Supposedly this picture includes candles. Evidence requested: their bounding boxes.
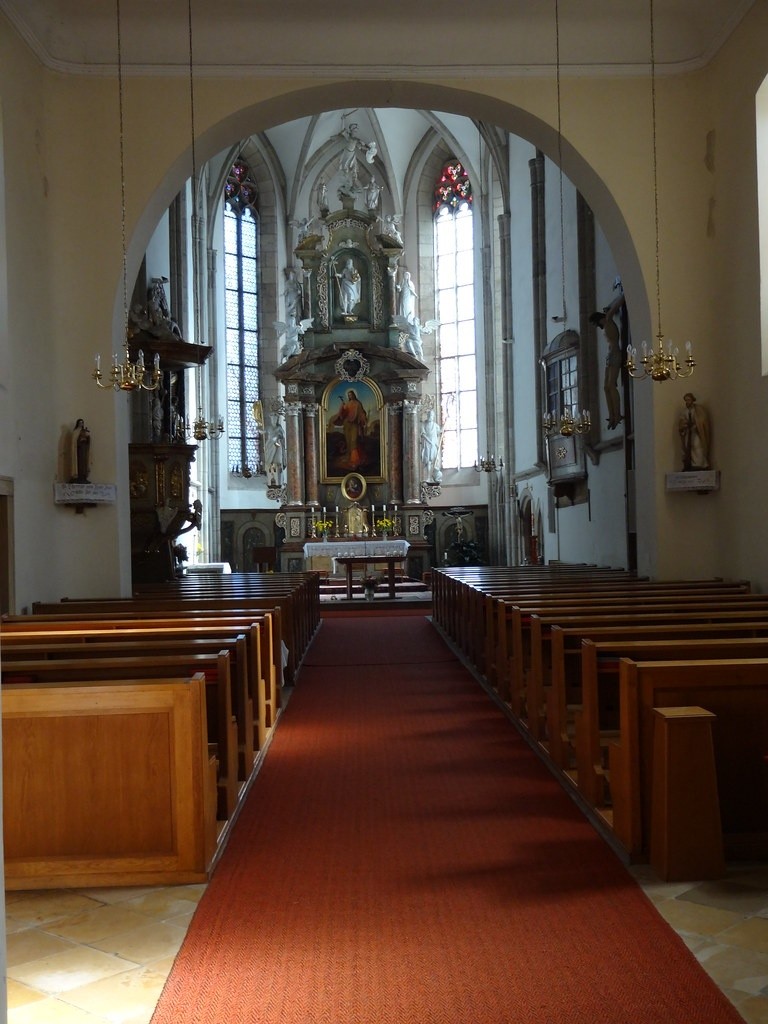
[372,505,374,511]
[336,506,339,512]
[383,505,386,511]
[323,507,326,512]
[395,505,397,510]
[312,507,314,512]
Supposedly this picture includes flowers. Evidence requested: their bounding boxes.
[358,574,379,588]
[376,518,392,531]
[315,521,333,536]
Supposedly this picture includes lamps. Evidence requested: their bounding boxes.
[527,0,590,441]
[624,0,696,381]
[94,0,157,389]
[474,130,505,472]
[172,0,225,444]
[232,143,259,479]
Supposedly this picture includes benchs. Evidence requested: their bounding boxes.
[3,571,320,892]
[429,563,768,866]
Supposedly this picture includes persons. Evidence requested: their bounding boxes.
[589,294,624,430]
[281,272,305,360]
[335,258,361,314]
[395,271,430,363]
[70,418,91,480]
[266,411,285,487]
[421,410,442,484]
[678,392,712,471]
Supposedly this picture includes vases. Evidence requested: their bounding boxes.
[382,532,388,541]
[364,588,374,601]
[322,536,328,543]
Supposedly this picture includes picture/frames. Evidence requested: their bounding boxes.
[319,375,389,485]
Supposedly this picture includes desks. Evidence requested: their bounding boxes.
[335,556,405,599]
[303,540,410,583]
